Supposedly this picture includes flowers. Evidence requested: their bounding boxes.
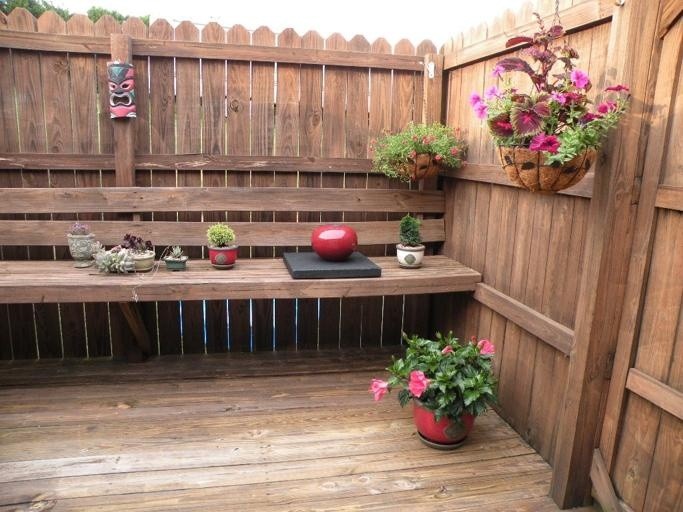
[366,118,474,191]
[367,323,506,442]
[466,1,646,173]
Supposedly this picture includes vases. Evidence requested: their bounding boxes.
[493,137,598,195]
[397,150,443,185]
[413,397,482,453]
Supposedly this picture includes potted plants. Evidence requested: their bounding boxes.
[60,219,241,277]
[394,211,427,270]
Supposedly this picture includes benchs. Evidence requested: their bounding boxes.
[0,174,482,307]
